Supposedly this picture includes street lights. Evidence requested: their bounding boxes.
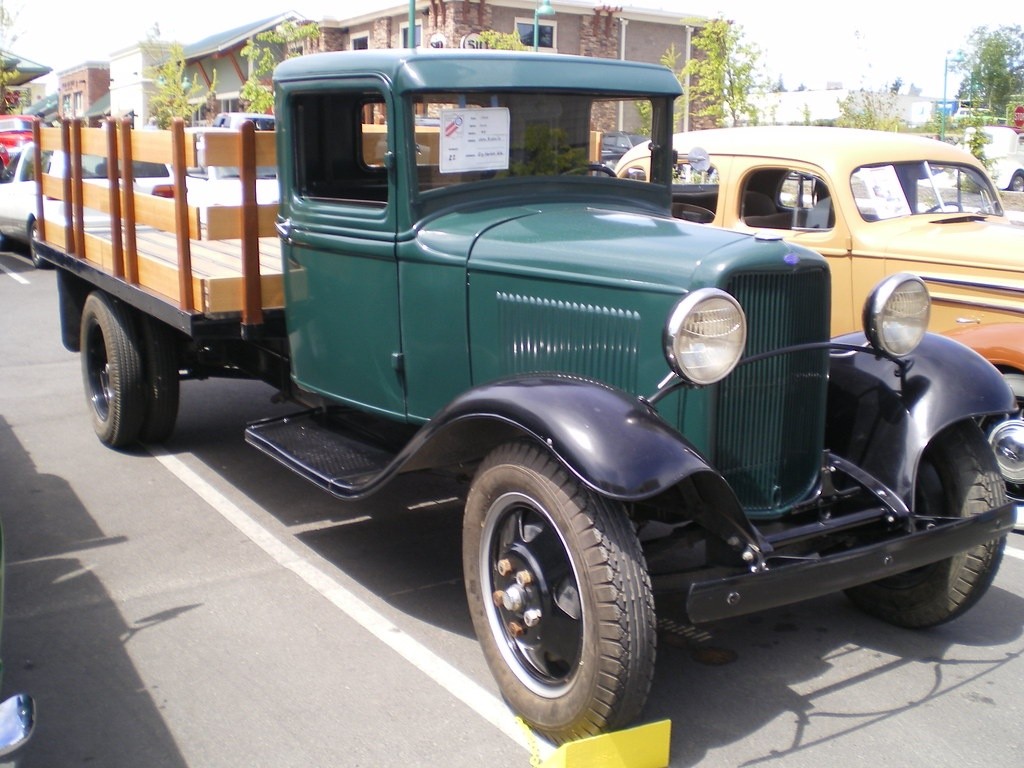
[532,1,554,53]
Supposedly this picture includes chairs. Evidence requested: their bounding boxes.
[744,190,777,215]
[815,174,882,229]
[673,191,716,217]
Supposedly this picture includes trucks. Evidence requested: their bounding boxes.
[23,44,1024,748]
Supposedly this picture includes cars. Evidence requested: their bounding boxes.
[615,124,1024,415]
[1,112,276,270]
[943,106,1023,192]
[597,130,655,180]
